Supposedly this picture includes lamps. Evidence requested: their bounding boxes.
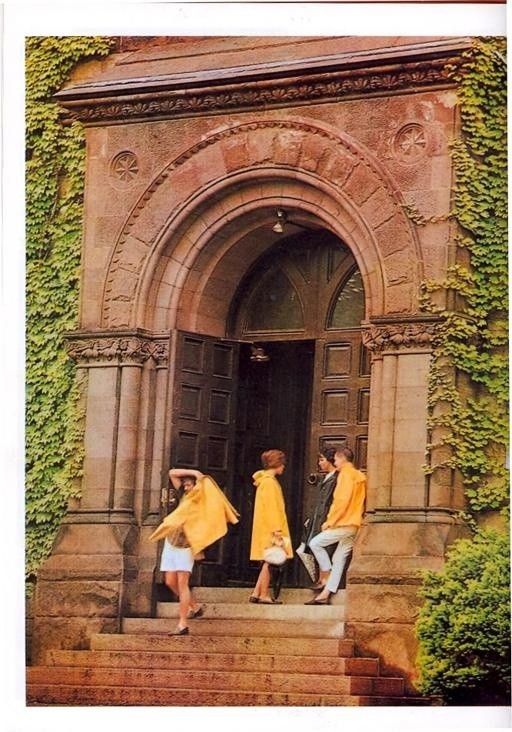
[272,208,289,233]
[246,345,270,363]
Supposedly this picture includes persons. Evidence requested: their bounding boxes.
[304,445,338,581]
[303,448,367,605]
[250,449,294,605]
[149,469,241,635]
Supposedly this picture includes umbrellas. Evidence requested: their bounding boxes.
[269,564,284,600]
[295,527,316,583]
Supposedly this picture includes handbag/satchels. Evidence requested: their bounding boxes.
[263,539,287,566]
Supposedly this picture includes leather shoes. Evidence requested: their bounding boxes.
[168,628,189,636]
[188,608,204,618]
[249,596,261,604]
[304,579,330,605]
[260,598,282,604]
